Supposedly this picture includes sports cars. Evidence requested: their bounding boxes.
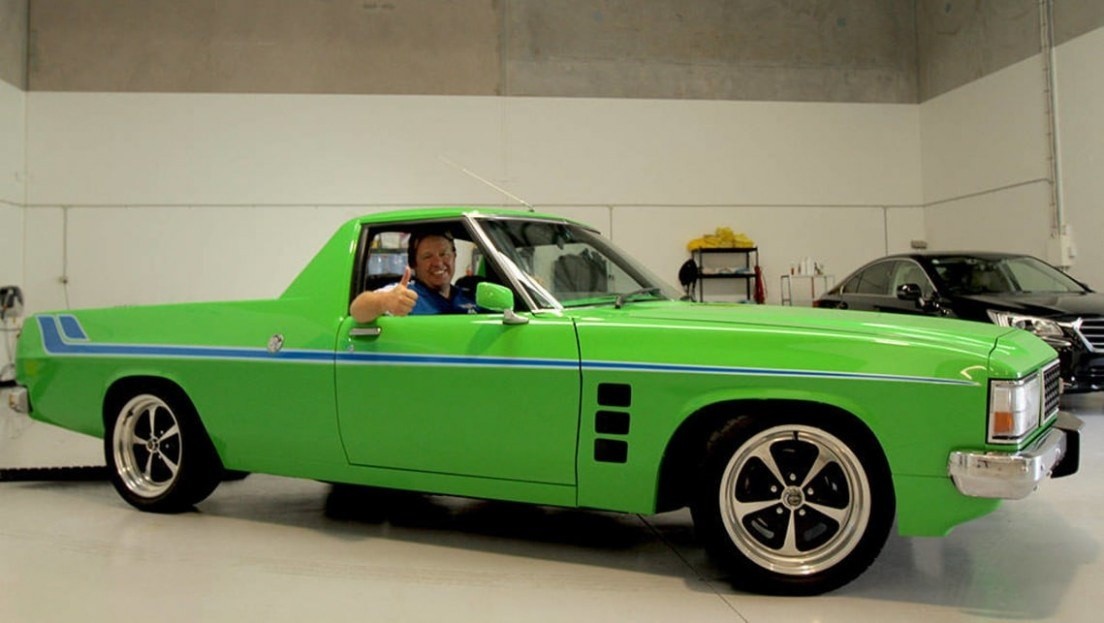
[5,207,1069,598]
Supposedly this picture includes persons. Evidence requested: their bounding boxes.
[350,226,500,324]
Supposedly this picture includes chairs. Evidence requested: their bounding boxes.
[457,275,489,302]
[972,268,1010,293]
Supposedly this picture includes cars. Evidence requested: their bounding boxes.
[810,250,1104,397]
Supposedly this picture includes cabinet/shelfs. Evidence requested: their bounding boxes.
[0,285,24,388]
[690,247,760,304]
[780,274,835,307]
